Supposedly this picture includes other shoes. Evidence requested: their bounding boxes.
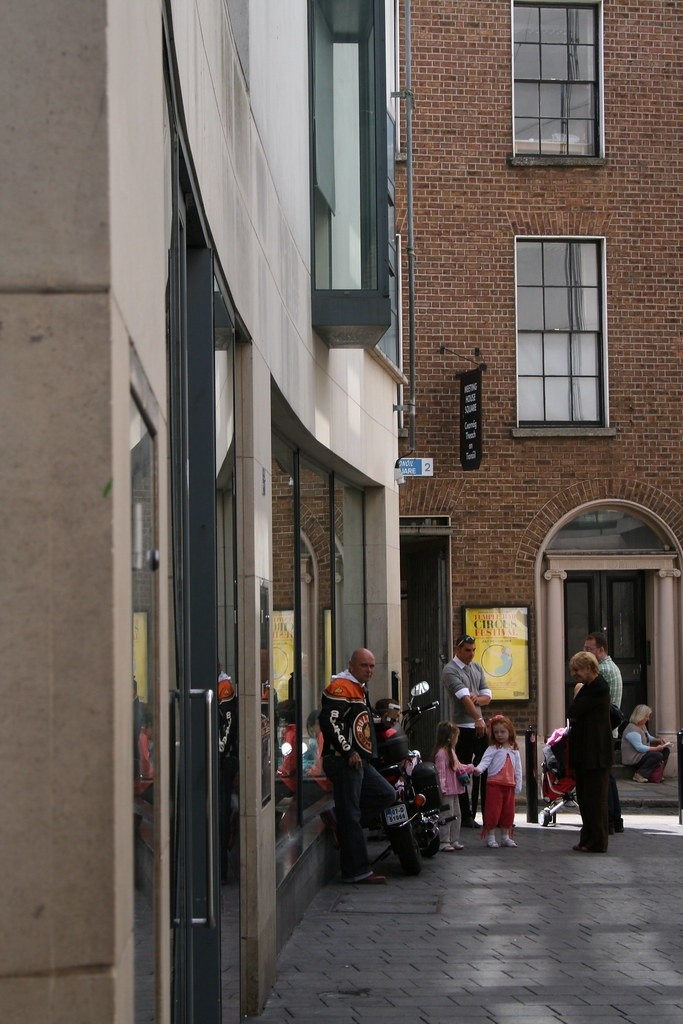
[502,839,518,847]
[607,829,615,835]
[443,846,455,851]
[461,820,481,828]
[452,843,464,848]
[488,841,499,847]
[616,827,624,833]
[633,773,648,783]
[573,844,589,852]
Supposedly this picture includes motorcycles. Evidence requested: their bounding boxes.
[373,680,458,875]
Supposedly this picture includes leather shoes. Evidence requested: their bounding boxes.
[320,810,340,851]
[358,872,386,884]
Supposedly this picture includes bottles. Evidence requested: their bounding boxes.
[456,767,472,787]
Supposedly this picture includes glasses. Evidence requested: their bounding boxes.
[457,636,475,647]
[645,732,649,743]
[583,647,598,652]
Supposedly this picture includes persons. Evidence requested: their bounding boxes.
[566,631,625,855]
[621,705,671,784]
[133,677,155,827]
[275,700,297,807]
[435,633,529,853]
[216,656,241,885]
[319,647,396,885]
[303,710,332,811]
[374,698,402,723]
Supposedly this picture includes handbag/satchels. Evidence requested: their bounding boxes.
[649,761,665,783]
[609,703,625,730]
